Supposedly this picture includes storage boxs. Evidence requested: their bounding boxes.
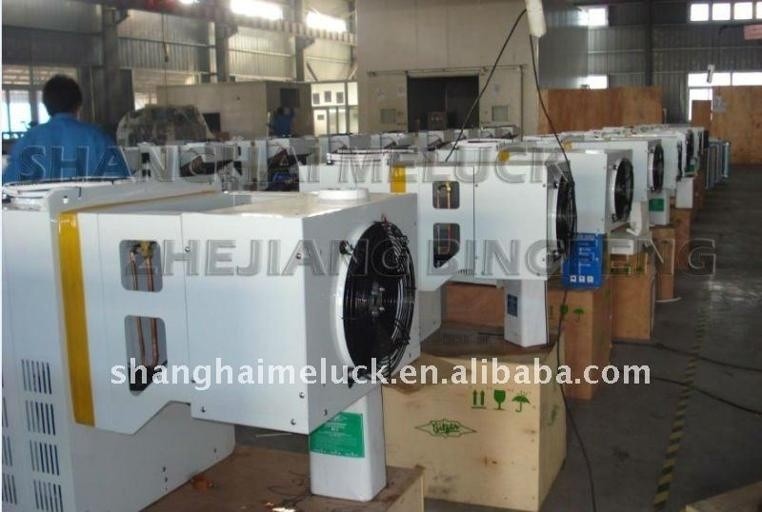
[383,334,568,512]
[441,279,613,400]
[137,445,423,511]
[611,203,691,343]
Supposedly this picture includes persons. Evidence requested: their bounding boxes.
[271,105,294,137]
[2,76,129,184]
